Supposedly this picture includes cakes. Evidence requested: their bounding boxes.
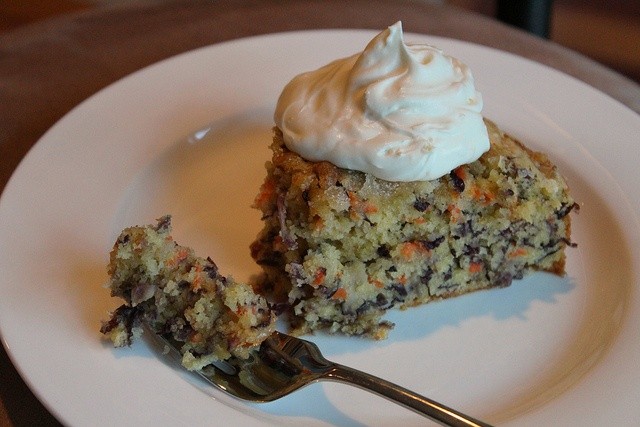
[249,20,580,340]
[98,215,278,371]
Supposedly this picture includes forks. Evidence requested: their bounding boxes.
[139,325,489,423]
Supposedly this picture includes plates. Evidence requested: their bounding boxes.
[1,27,635,426]
